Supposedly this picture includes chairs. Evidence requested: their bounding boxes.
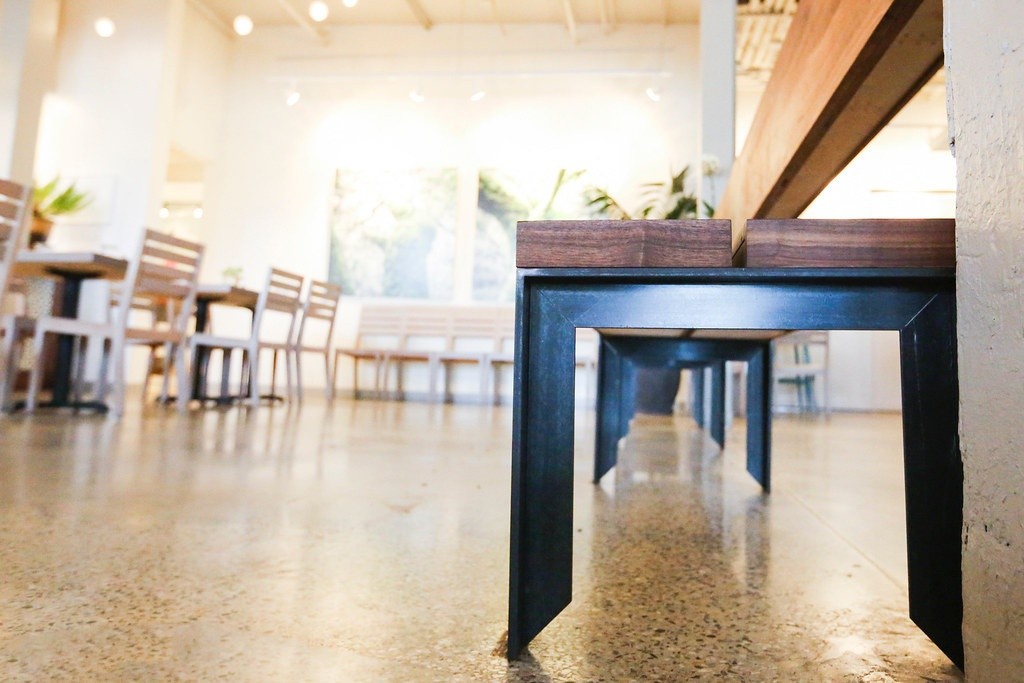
[777,331,827,415]
[0,178,586,422]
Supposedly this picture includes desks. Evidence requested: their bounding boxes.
[189,286,258,408]
[11,251,128,414]
